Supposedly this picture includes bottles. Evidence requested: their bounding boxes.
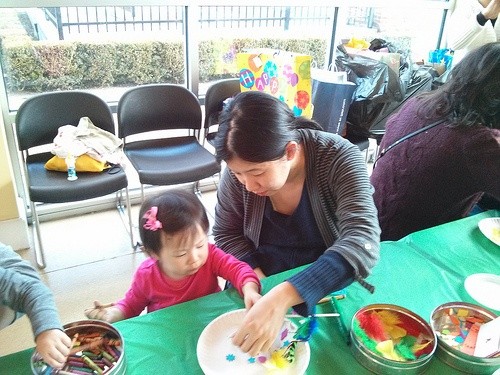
[65,151,78,181]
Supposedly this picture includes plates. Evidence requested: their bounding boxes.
[463,272,500,311]
[478,217,500,246]
[197,309,310,375]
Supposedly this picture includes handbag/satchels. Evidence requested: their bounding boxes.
[234,46,315,119]
[308,59,356,134]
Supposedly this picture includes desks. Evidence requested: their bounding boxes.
[1,204,500,375]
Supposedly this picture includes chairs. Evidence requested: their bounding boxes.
[14,78,243,270]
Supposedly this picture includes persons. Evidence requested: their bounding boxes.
[85,188,263,323]
[212,91,380,355]
[0,241,72,369]
[448,0,500,72]
[370,41,500,242]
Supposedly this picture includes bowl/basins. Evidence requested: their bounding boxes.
[31,318,127,375]
[349,303,438,375]
[430,301,500,375]
